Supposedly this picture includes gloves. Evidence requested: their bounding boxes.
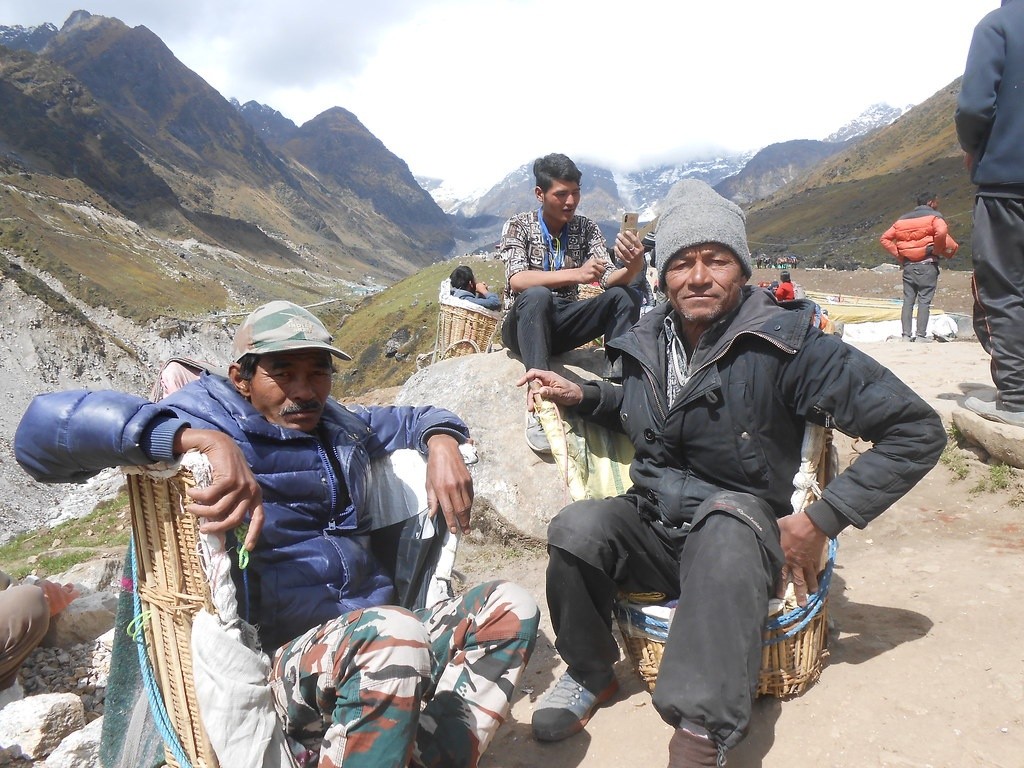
[34,579,79,616]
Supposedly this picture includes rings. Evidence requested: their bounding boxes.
[629,247,635,251]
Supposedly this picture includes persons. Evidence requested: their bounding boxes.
[880,192,959,342]
[0,572,78,690]
[607,247,668,314]
[955,0,1024,427]
[757,254,797,269]
[517,178,948,768]
[450,266,501,310]
[500,153,645,454]
[768,271,797,301]
[15,299,540,768]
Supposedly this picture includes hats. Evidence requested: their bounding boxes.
[231,299,352,361]
[654,177,752,291]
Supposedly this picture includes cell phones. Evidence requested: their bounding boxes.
[620,213,638,245]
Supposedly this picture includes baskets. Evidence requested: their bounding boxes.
[436,295,502,357]
[614,561,828,697]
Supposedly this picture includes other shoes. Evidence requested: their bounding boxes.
[915,335,934,343]
[903,335,910,338]
[964,386,1024,427]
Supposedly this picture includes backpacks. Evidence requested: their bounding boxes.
[792,281,803,299]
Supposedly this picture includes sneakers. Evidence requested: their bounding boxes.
[525,406,552,455]
[532,664,620,742]
